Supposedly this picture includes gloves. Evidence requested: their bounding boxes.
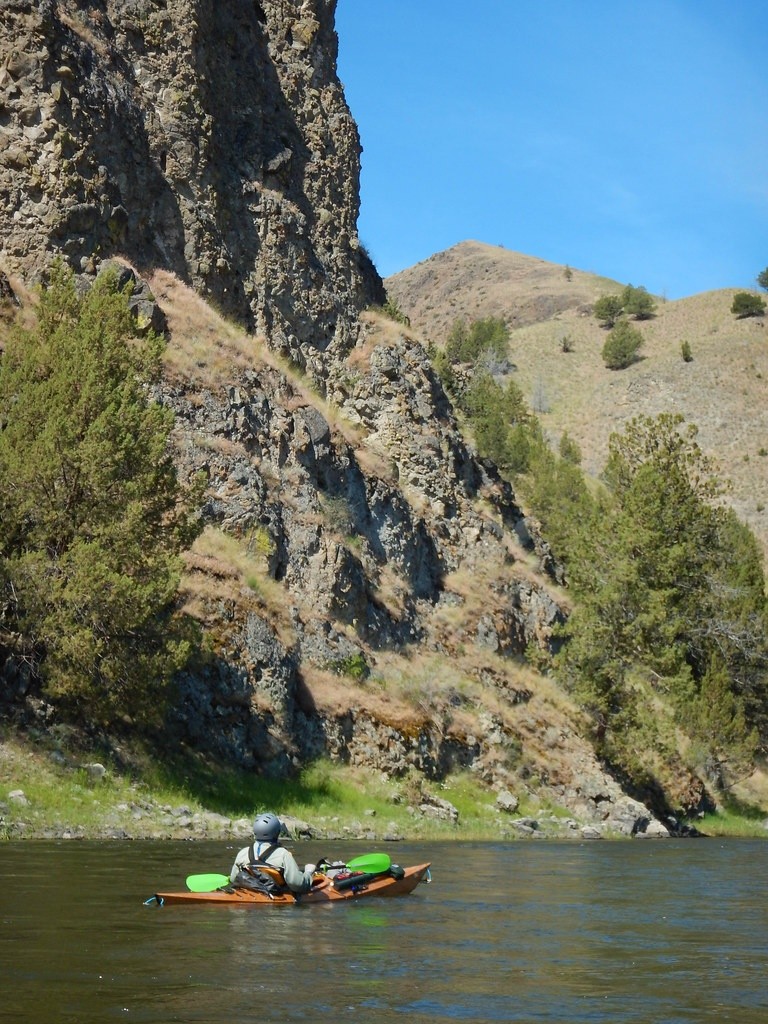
[304,863,317,874]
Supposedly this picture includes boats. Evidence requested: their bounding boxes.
[154,861,433,906]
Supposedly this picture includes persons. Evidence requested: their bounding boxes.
[230,813,317,893]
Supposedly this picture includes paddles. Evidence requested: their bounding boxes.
[185,853,391,893]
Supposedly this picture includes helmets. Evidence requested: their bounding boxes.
[253,813,280,840]
